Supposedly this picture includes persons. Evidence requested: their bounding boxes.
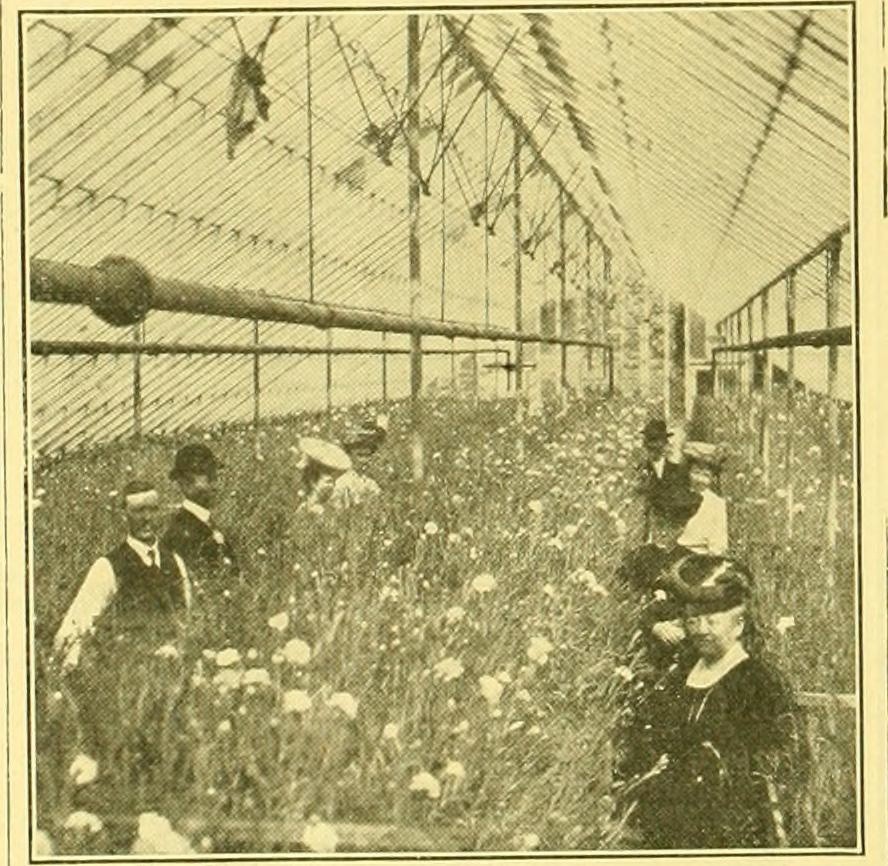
[54,436,380,665]
[630,419,809,849]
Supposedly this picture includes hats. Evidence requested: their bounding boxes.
[682,441,722,465]
[671,550,746,601]
[168,444,224,476]
[641,419,675,438]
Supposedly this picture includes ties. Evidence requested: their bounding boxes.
[149,551,161,573]
